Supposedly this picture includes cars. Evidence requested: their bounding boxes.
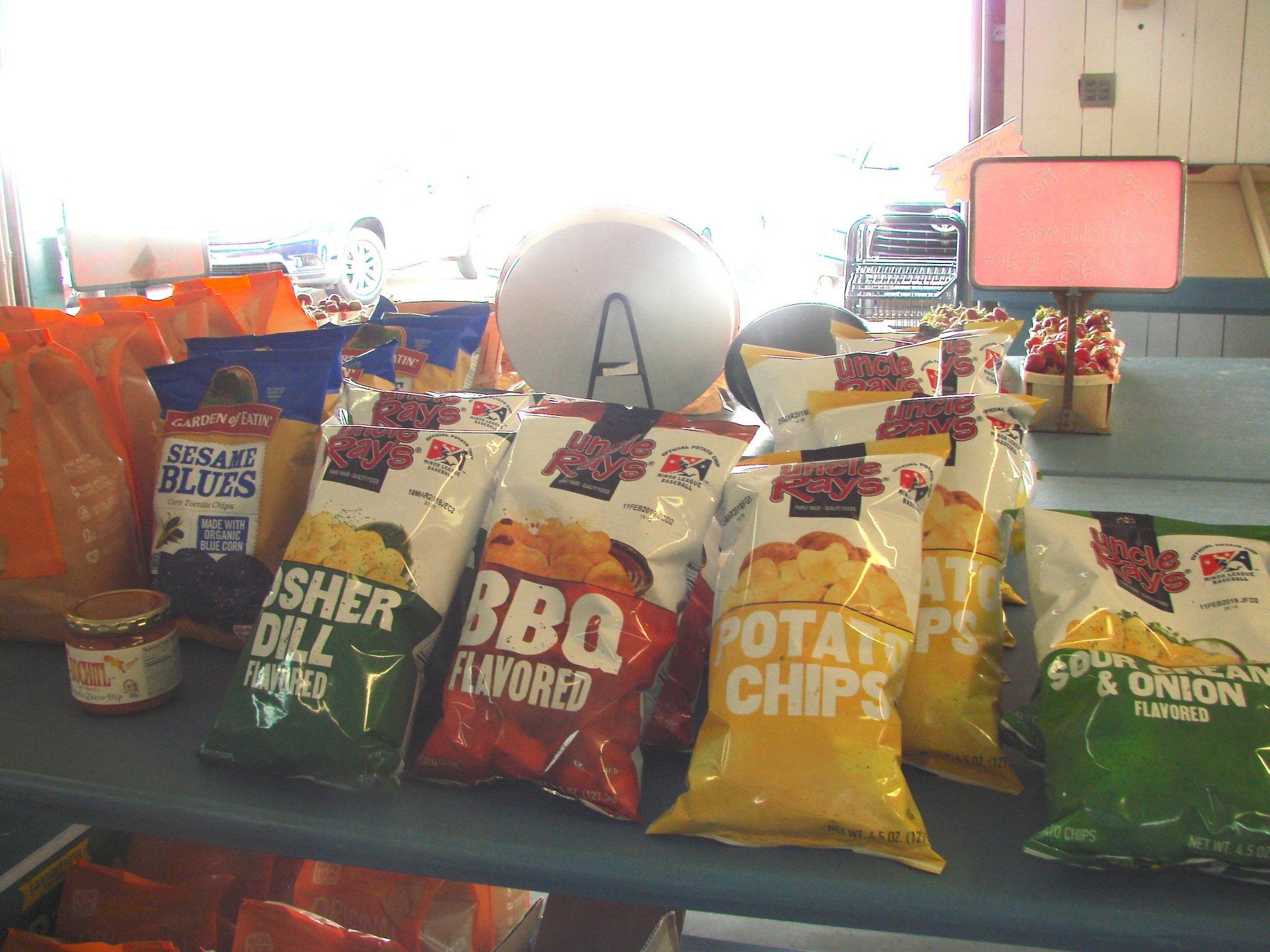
[27,168,511,309]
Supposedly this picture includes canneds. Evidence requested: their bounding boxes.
[62,588,183,715]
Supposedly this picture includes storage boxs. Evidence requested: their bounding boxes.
[1023,339,1125,436]
[492,890,687,952]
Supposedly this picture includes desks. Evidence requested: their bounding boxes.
[0,474,1269,952]
[682,355,1270,482]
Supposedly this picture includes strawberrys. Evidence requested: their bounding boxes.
[924,303,1009,330]
[1023,306,1121,375]
